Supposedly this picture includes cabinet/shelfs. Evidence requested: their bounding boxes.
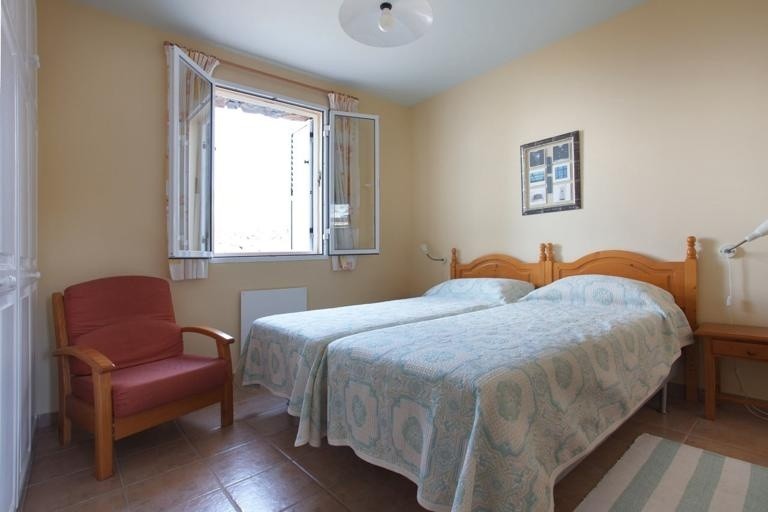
[0,3,37,512]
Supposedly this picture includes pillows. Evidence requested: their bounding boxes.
[524,274,676,310]
[420,275,533,304]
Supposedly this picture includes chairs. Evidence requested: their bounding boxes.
[49,274,238,479]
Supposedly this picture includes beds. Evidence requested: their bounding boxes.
[238,241,544,454]
[326,234,697,512]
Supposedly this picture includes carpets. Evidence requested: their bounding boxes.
[567,431,767,512]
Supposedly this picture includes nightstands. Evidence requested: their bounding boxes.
[692,321,766,421]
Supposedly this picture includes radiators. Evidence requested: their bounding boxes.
[240,286,309,356]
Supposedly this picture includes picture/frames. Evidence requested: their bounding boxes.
[520,128,582,216]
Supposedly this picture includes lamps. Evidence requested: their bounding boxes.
[339,0,432,49]
